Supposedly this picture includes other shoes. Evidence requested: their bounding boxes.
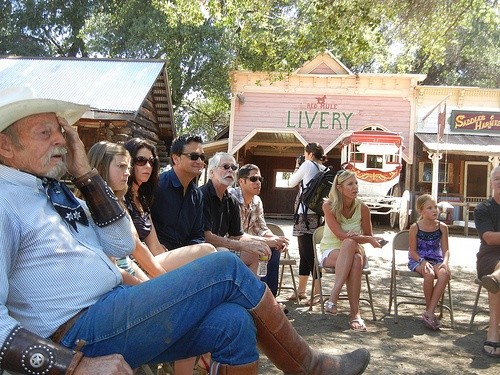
[286,293,307,301]
[305,297,323,306]
[196,352,211,374]
[423,314,442,330]
[279,304,289,315]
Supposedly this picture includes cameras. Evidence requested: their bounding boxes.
[298,155,305,165]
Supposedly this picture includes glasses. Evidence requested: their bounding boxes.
[181,152,205,160]
[215,164,238,170]
[135,156,158,166]
[242,176,263,182]
[336,170,345,185]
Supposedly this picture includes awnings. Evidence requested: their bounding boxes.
[414,133,500,157]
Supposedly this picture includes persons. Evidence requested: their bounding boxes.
[285,141,332,306]
[472,167,500,358]
[0,106,371,375]
[320,170,384,332]
[73,135,289,375]
[407,193,451,332]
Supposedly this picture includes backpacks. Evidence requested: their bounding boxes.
[300,160,337,216]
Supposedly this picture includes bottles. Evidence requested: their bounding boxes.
[256,251,269,277]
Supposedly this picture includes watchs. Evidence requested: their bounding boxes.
[419,258,425,265]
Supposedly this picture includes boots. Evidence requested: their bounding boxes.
[247,282,371,375]
[210,358,261,375]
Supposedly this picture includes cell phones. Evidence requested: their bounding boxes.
[379,240,389,246]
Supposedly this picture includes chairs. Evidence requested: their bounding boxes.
[309,226,377,322]
[388,230,455,328]
[266,224,302,307]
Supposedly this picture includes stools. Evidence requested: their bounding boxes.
[470,279,492,332]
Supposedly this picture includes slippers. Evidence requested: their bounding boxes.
[348,318,366,332]
[480,275,500,294]
[483,337,500,358]
[325,300,338,315]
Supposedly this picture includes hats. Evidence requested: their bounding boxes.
[0,86,91,134]
